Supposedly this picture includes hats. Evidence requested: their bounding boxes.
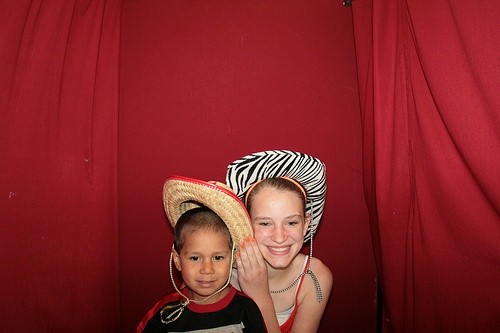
[226,150,327,242]
[163,175,255,270]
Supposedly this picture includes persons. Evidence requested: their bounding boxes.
[135,176,267,333]
[181,150,333,333]
